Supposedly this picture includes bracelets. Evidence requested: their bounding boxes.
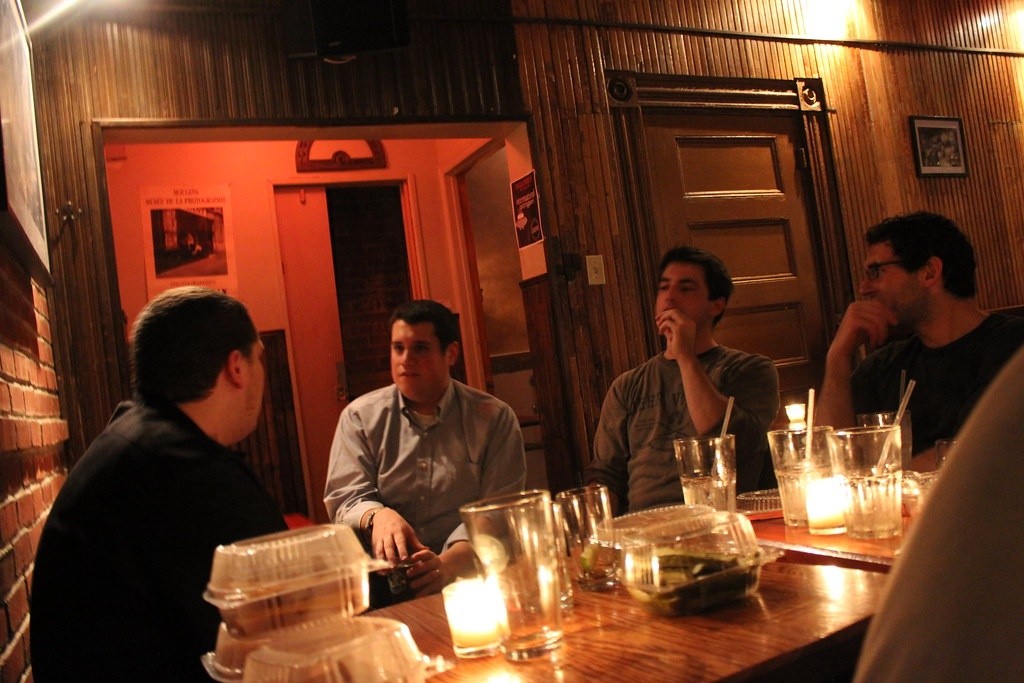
[366,507,387,535]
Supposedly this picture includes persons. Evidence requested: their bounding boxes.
[323,299,528,603]
[579,246,781,519]
[812,210,1024,475]
[28,285,290,683]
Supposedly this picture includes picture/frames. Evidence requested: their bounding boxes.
[908,114,970,179]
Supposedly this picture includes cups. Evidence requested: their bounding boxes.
[458,494,564,659]
[857,409,912,472]
[675,434,736,514]
[769,427,837,527]
[512,505,573,611]
[827,427,903,538]
[556,485,614,591]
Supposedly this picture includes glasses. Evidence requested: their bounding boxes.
[861,260,904,282]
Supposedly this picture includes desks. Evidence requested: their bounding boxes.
[749,513,912,575]
[352,562,889,683]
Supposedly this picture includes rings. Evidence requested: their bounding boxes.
[436,569,440,577]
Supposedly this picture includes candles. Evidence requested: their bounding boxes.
[804,487,848,535]
[441,574,509,657]
